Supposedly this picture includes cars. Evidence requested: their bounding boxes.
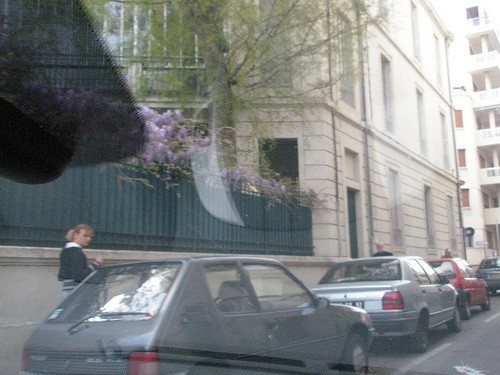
[21,256,374,375]
[311,256,462,353]
[422,258,492,319]
[477,259,500,295]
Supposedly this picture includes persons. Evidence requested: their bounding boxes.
[373,242,394,257]
[58,223,104,302]
[440,247,454,258]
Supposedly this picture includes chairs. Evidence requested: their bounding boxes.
[214,278,255,315]
[354,270,371,279]
